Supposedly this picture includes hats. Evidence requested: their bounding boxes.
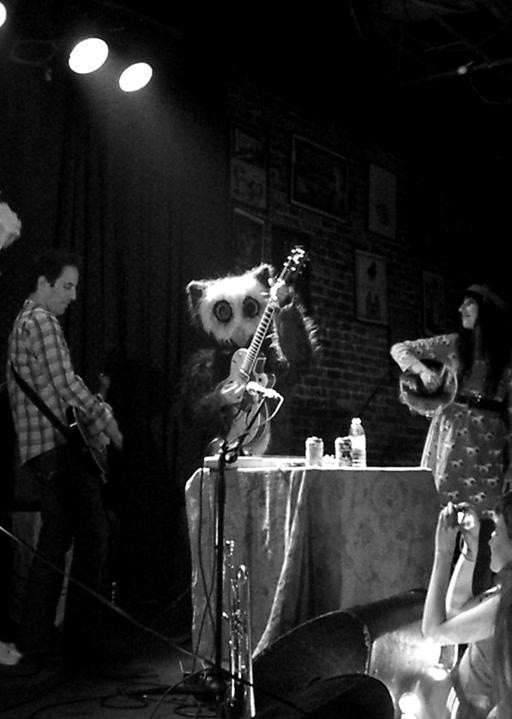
[464,279,503,314]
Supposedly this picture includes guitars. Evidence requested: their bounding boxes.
[213,244,311,447]
[65,374,111,478]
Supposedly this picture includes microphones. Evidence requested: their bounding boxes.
[246,381,281,399]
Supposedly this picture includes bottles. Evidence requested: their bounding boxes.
[348,417,367,467]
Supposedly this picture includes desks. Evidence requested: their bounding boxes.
[186,465,442,682]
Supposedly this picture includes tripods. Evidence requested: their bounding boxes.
[127,395,269,719]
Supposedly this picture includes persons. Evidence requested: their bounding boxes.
[369,493,512,719]
[389,281,509,597]
[7,252,154,683]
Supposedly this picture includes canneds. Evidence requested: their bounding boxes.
[335,437,352,464]
[304,437,324,464]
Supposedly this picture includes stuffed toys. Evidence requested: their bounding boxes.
[176,262,320,495]
